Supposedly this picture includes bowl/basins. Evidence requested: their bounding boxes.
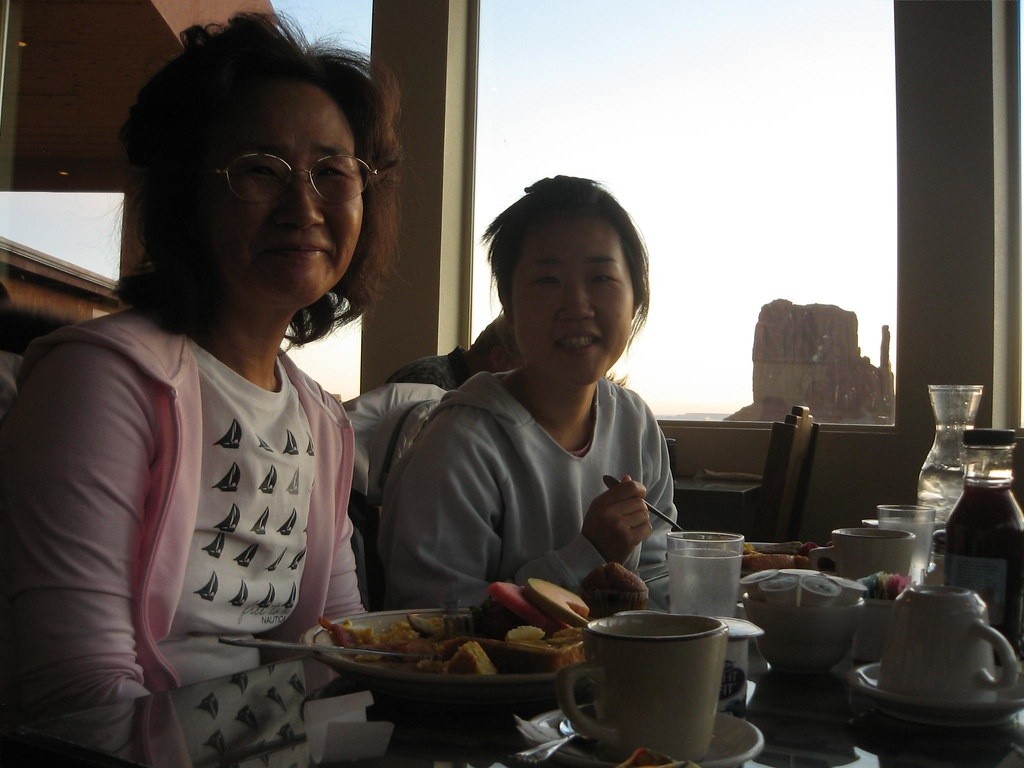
[742,593,866,675]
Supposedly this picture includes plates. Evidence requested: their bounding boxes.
[525,710,763,768]
[300,610,556,706]
[741,543,777,576]
[847,664,1024,727]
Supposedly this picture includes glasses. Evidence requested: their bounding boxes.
[202,154,379,203]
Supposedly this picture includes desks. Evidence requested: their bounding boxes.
[668,473,765,511]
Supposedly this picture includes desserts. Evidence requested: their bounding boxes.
[577,562,650,619]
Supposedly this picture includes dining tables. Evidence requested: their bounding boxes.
[0,553,1024,768]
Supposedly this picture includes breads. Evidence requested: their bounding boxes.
[445,640,498,676]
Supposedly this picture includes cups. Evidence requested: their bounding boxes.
[558,615,729,760]
[877,504,935,586]
[878,584,1018,700]
[810,527,917,581]
[717,617,763,719]
[666,531,744,618]
[852,598,895,655]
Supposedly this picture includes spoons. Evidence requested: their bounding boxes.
[510,705,598,762]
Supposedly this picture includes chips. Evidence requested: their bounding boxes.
[502,625,547,642]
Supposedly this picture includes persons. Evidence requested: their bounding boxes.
[342,173,679,612]
[0,10,394,721]
[42,656,341,768]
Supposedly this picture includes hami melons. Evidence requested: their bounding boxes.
[525,576,595,628]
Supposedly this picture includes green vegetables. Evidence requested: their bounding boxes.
[469,595,526,639]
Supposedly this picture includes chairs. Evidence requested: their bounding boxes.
[749,407,821,543]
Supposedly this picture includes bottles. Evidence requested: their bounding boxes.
[924,527,947,585]
[945,429,1024,666]
[918,385,985,527]
[665,438,676,482]
